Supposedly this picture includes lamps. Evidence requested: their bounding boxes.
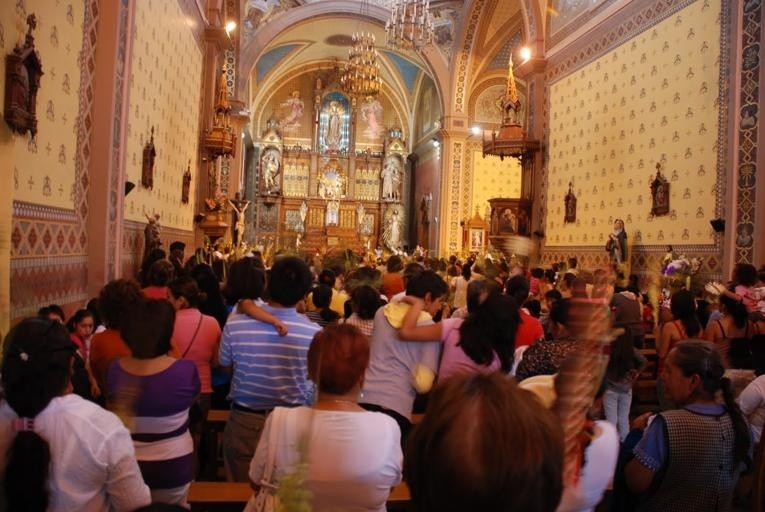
[338,0,385,104]
[382,0,440,53]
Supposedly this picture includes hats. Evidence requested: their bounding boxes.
[2,316,79,352]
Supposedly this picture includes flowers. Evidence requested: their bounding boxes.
[661,244,698,283]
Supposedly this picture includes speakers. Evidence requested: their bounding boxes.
[196,213,205,223]
[125,181,135,196]
[533,231,544,238]
[710,218,725,232]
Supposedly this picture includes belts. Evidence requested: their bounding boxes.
[232,401,272,415]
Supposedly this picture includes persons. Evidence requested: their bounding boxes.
[279,90,304,127]
[324,106,342,146]
[145,213,163,232]
[144,218,163,248]
[361,95,384,135]
[605,219,628,281]
[228,199,250,247]
[2,245,765,512]
[391,209,399,241]
[391,167,400,201]
[261,152,276,188]
[380,164,393,199]
